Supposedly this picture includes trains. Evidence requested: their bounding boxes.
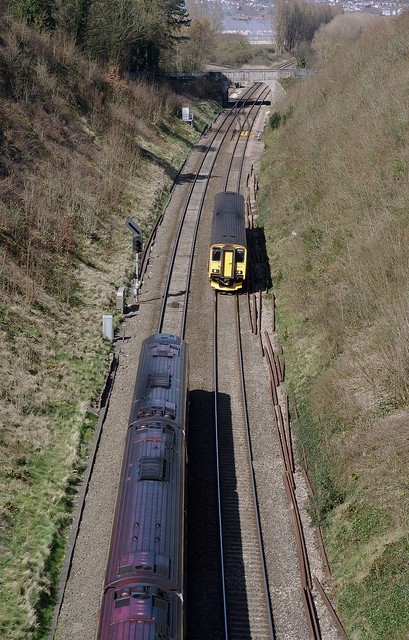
[209,191,247,294]
[98,334,188,640]
